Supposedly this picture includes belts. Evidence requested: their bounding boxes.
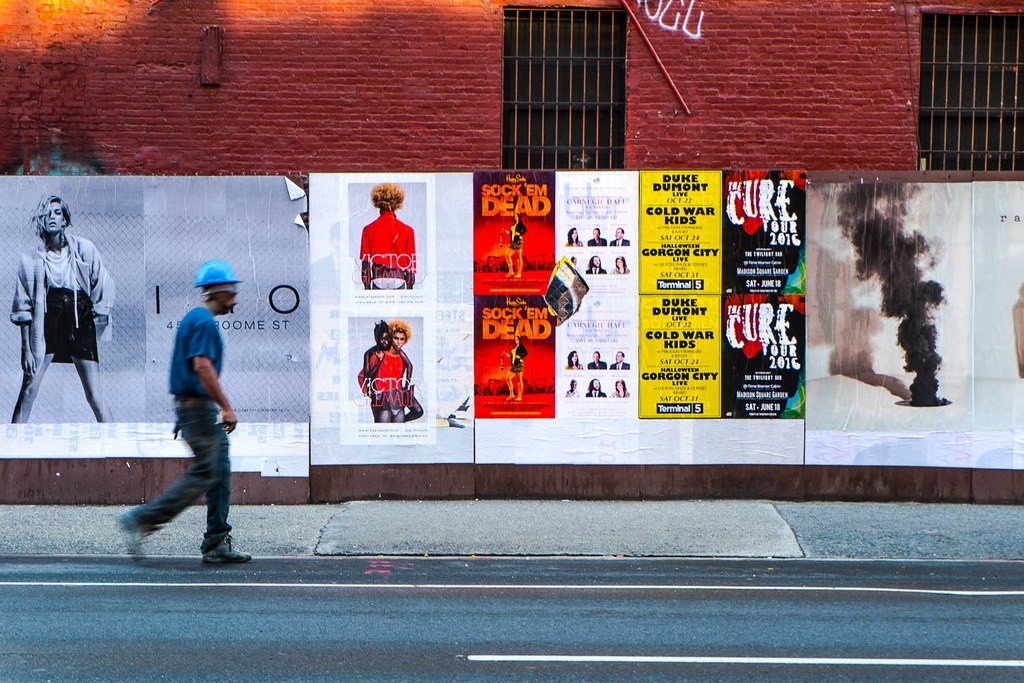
[174,398,215,408]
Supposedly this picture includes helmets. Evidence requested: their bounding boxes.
[194,260,245,287]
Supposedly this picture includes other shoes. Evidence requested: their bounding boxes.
[201,535,251,563]
[118,511,162,564]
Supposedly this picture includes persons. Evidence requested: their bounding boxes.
[357,319,423,423]
[501,212,630,402]
[120,258,251,565]
[9,195,116,424]
[359,183,417,290]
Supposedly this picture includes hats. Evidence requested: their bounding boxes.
[197,283,239,301]
